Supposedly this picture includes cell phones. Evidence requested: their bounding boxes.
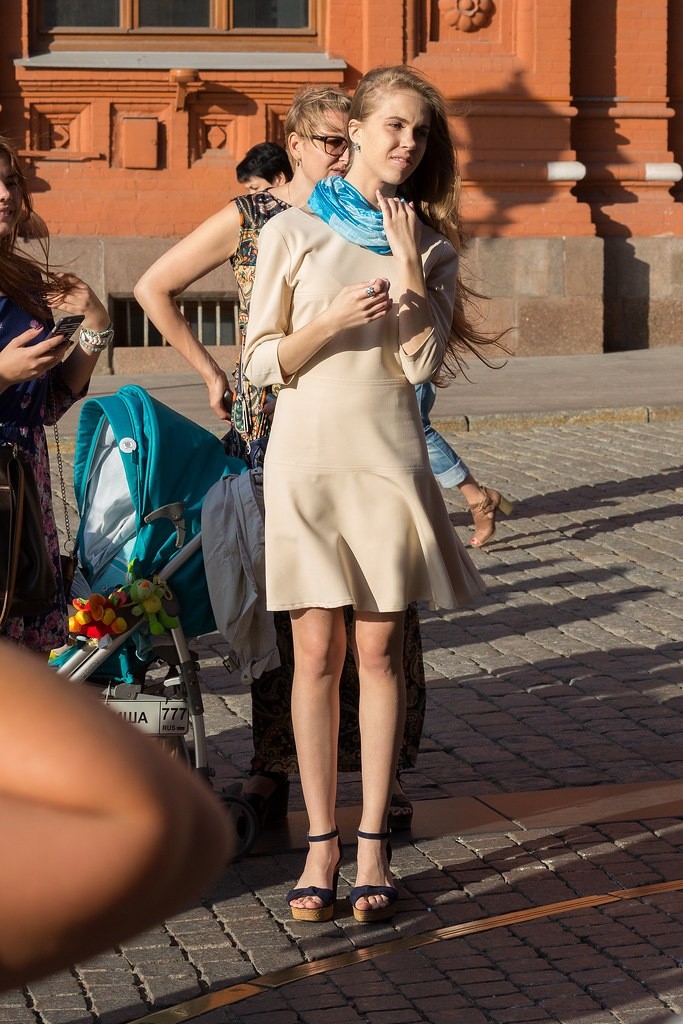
[43,314,85,342]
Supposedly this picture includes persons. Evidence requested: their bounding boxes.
[241,66,488,921]
[0,138,115,652]
[236,143,514,547]
[133,86,427,837]
[0,639,236,991]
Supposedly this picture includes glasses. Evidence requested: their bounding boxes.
[300,134,349,156]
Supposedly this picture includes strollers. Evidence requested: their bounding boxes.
[44,384,257,859]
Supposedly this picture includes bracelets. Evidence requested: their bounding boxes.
[78,322,114,353]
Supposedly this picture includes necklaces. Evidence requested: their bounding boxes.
[288,183,293,206]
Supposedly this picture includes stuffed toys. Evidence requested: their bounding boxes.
[67,557,180,641]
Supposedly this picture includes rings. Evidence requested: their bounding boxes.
[366,286,374,297]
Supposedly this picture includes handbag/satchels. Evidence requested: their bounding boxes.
[0,449,50,633]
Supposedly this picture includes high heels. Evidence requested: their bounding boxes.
[387,772,414,830]
[350,828,399,922]
[469,487,513,547]
[285,827,343,921]
[227,770,290,822]
